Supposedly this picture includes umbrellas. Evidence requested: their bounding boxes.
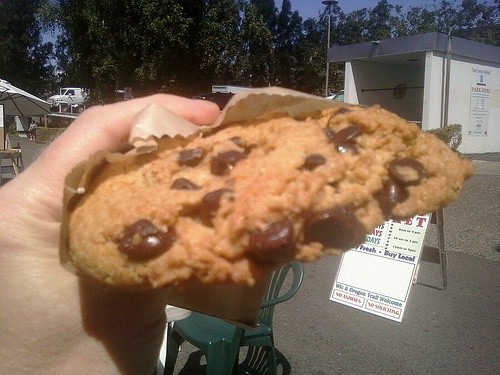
[0,80,52,150]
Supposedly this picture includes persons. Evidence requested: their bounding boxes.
[0,92,220,375]
[27,119,37,142]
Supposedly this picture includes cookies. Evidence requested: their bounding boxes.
[65,102,469,289]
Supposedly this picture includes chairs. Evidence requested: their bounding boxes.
[163,261,304,375]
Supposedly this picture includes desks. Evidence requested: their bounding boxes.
[0,152,25,187]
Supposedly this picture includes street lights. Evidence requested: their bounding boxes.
[322,0,338,98]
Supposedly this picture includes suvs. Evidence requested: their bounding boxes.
[48,87,84,112]
